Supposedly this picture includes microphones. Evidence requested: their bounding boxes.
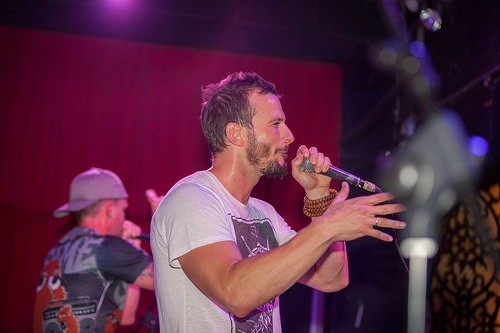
[129,232,150,240]
[299,156,382,194]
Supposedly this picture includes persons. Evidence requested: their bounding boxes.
[32,167,167,333]
[150,71,410,333]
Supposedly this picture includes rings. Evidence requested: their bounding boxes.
[375,217,380,226]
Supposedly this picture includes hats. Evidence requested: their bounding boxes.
[54,167,129,220]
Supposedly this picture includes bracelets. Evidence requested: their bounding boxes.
[303,188,341,218]
